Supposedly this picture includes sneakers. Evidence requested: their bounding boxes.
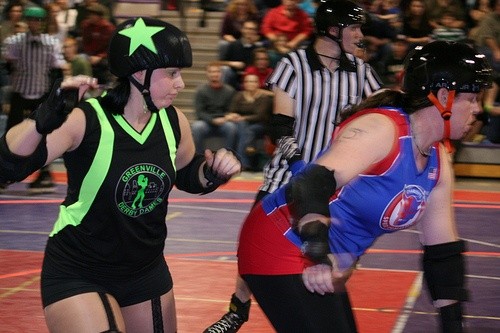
[202,313,244,333]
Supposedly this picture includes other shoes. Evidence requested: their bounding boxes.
[29,171,56,193]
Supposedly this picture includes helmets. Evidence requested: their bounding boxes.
[401,40,493,91]
[315,0,365,32]
[108,16,193,77]
[23,7,48,21]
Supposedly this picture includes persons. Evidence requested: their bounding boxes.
[201,0,385,333]
[0,0,500,191]
[0,16,242,333]
[232,36,497,333]
